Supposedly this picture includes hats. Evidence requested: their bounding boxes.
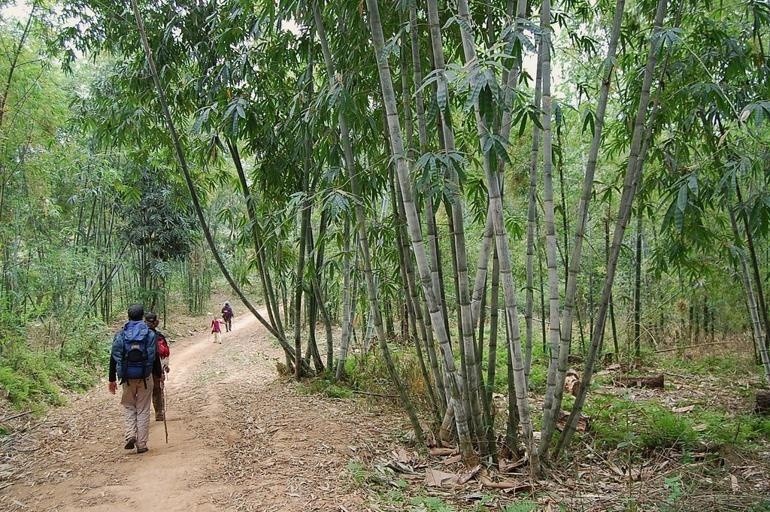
[145,314,157,323]
[128,304,144,319]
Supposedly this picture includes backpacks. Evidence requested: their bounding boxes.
[120,325,150,379]
[223,307,231,317]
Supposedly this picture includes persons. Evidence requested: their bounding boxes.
[210,314,227,344]
[221,302,234,332]
[145,312,171,422]
[108,305,160,453]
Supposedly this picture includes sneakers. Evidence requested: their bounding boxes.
[124,437,137,449]
[213,341,221,344]
[226,329,231,332]
[156,413,164,421]
[137,448,149,453]
